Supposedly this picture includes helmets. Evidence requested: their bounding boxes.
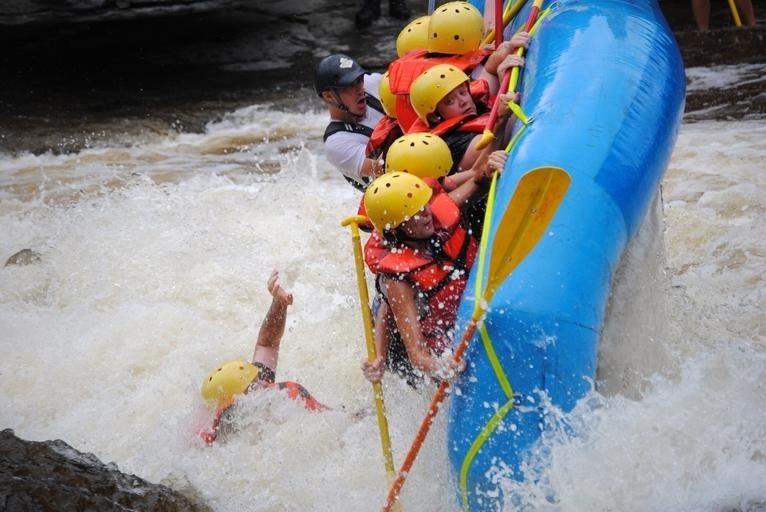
[314,52,365,98]
[363,0,484,236]
[201,359,259,415]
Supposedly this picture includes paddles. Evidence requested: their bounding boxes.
[382,165,572,512]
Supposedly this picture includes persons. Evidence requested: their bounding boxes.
[313,0,534,385]
[692,0,757,32]
[200,269,337,446]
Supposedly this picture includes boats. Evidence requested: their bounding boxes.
[446,0,686,512]
